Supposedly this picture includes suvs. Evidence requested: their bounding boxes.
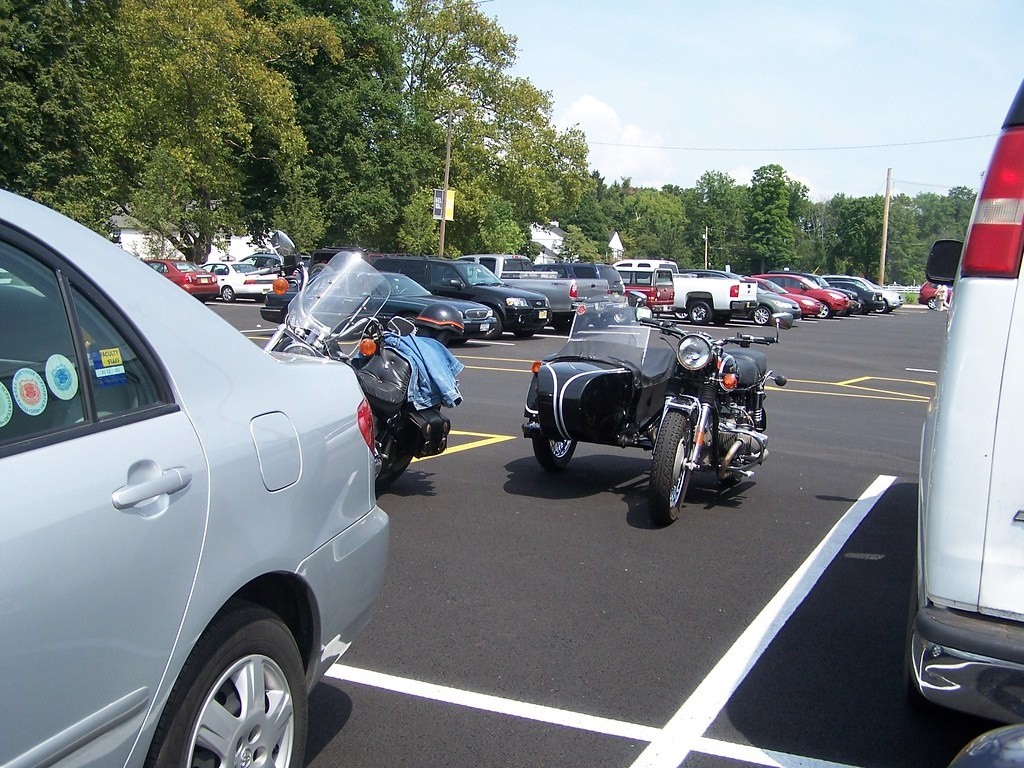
[364,255,555,347]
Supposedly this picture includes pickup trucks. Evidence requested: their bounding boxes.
[453,252,615,327]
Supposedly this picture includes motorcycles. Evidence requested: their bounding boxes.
[517,288,794,526]
[245,228,462,490]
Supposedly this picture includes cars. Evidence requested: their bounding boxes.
[0,184,402,766]
[918,280,953,311]
[146,259,222,308]
[259,269,500,350]
[527,259,909,329]
[193,262,284,303]
